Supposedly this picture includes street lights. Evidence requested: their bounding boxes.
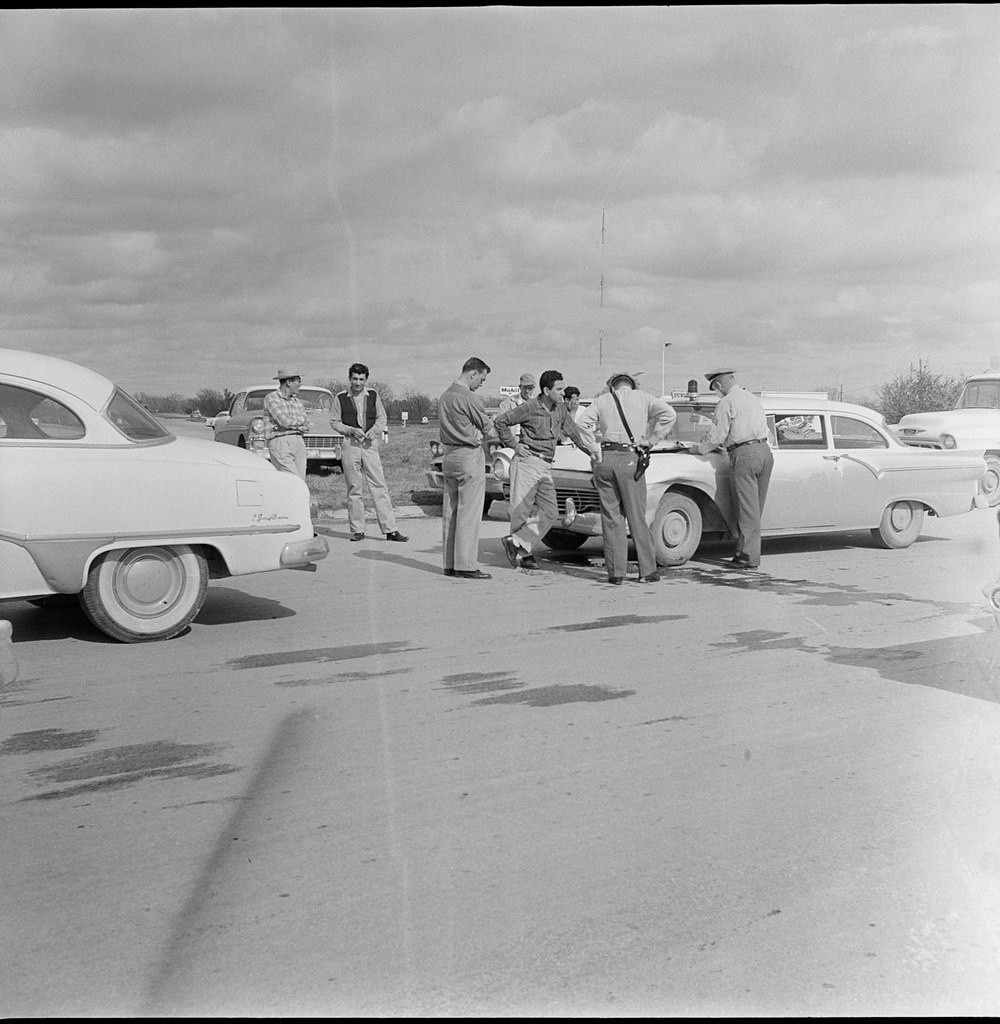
[660,341,676,398]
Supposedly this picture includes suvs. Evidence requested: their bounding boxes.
[870,373,1000,510]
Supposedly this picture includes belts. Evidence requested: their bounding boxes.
[268,433,303,441]
[728,439,767,453]
[532,453,552,464]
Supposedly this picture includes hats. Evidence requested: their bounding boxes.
[519,374,536,385]
[704,370,736,391]
[272,365,305,380]
[607,372,639,391]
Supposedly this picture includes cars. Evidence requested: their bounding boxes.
[0,347,332,643]
[426,384,988,568]
[190,411,202,418]
[205,411,230,430]
[213,385,345,471]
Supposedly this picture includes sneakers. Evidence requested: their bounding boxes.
[517,547,539,569]
[501,535,519,568]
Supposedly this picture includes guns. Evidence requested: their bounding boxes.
[634,449,650,482]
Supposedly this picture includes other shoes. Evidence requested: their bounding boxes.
[608,577,624,584]
[638,573,660,583]
[724,559,758,571]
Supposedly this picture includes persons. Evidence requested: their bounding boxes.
[499,373,537,444]
[690,368,774,570]
[576,371,676,585]
[263,367,311,480]
[493,370,596,570]
[437,357,495,578]
[330,363,410,542]
[557,386,587,446]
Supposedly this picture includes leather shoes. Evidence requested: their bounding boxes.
[350,532,364,541]
[387,531,408,542]
[444,568,491,580]
